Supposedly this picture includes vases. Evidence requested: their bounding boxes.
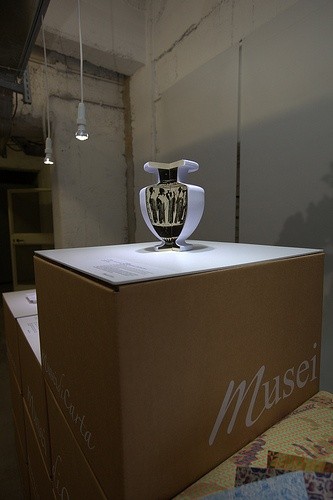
[139,161,203,252]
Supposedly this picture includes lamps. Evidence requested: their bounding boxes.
[74,103,88,140]
[43,137,53,164]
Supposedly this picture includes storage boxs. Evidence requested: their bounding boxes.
[2,237,324,500]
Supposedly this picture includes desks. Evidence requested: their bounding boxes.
[168,389,333,499]
